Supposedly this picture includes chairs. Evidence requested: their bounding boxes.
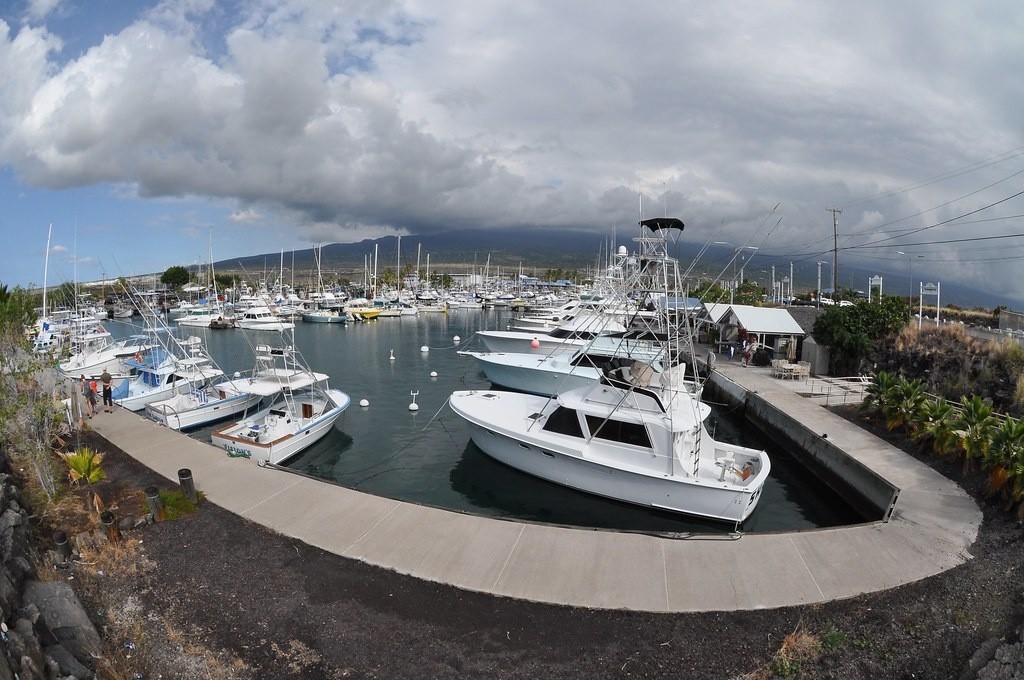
[771,358,811,381]
[730,466,750,480]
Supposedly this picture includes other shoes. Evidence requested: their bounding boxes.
[104,410,109,412]
[92,411,95,413]
[96,412,98,414]
[110,411,112,413]
[89,416,92,419]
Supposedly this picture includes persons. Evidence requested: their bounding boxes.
[531,337,539,348]
[646,303,654,310]
[101,370,113,414]
[728,338,757,360]
[80,374,93,419]
[89,377,98,414]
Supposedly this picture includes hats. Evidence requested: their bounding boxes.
[103,367,107,371]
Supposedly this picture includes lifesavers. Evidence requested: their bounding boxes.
[135,352,142,363]
[706,352,716,364]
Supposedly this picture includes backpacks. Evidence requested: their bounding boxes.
[82,382,92,398]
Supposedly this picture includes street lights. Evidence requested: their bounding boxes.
[819,259,835,293]
[713,242,758,296]
[897,251,925,316]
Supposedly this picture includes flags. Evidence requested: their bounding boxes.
[135,352,142,364]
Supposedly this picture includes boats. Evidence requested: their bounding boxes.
[278,423,354,485]
[24,192,785,527]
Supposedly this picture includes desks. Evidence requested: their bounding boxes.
[783,364,802,380]
